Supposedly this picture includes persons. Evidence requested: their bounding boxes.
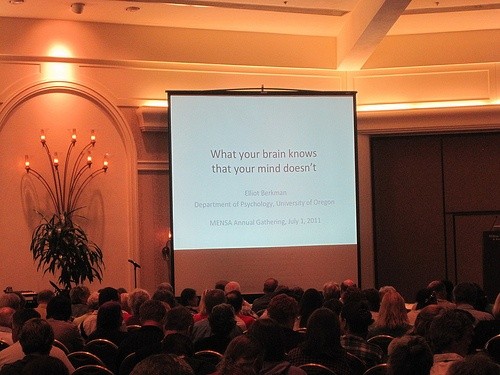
[0,277,500,375]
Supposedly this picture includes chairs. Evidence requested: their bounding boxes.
[482,334,500,365]
[345,351,367,368]
[84,338,119,372]
[297,362,336,375]
[66,350,107,369]
[126,324,142,333]
[70,365,115,375]
[366,334,395,357]
[194,349,224,367]
[119,350,135,375]
[51,338,70,355]
[361,361,388,375]
[295,327,308,339]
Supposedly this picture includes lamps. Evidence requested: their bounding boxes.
[25,126,110,228]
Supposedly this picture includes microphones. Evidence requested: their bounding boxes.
[128,259,141,268]
